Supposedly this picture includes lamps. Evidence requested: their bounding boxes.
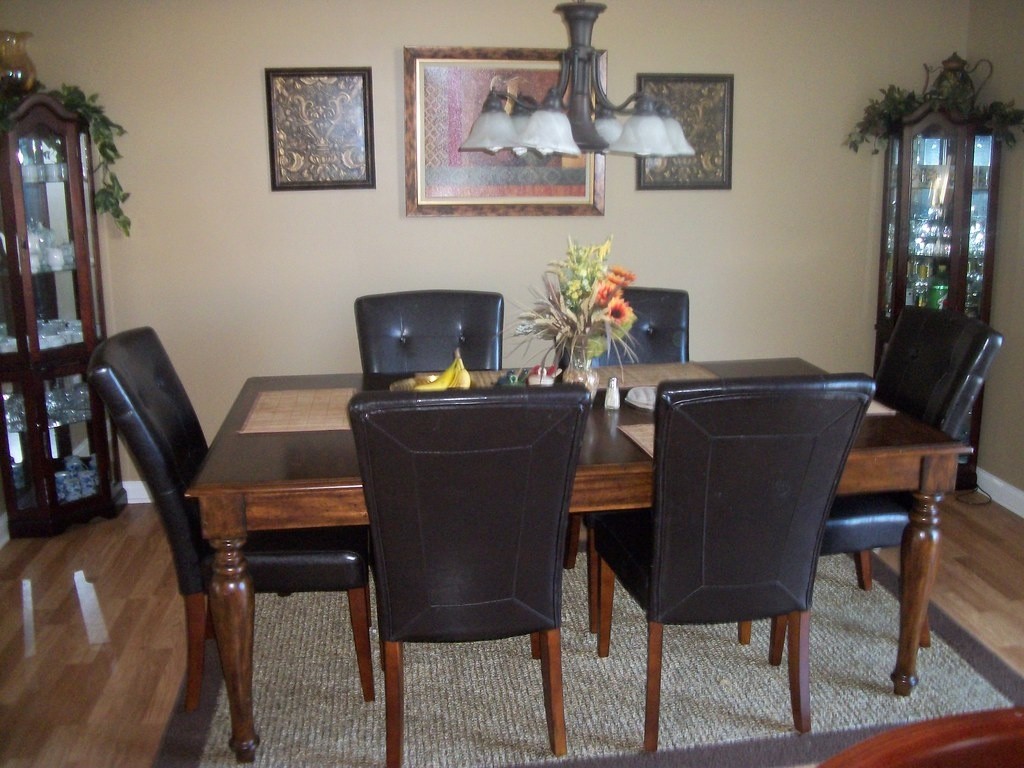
[456,0,697,159]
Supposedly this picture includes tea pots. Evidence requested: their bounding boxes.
[922,51,994,121]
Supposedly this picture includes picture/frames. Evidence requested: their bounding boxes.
[402,44,607,217]
[264,66,376,192]
[635,71,733,191]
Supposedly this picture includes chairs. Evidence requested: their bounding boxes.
[353,288,504,375]
[739,304,1007,664]
[87,325,375,711]
[546,284,692,569]
[588,372,876,756]
[343,383,590,767]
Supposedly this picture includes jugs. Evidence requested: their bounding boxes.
[0,219,54,265]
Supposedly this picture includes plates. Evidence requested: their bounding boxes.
[389,375,475,391]
[625,387,657,410]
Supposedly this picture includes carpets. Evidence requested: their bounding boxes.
[151,538,1024,768]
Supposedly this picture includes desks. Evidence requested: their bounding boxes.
[183,356,973,762]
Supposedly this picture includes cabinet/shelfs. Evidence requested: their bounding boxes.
[871,99,1004,493]
[0,90,127,537]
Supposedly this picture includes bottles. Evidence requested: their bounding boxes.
[886,258,983,319]
[605,377,620,409]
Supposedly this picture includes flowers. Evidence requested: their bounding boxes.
[493,231,642,381]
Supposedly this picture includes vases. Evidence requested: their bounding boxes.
[0,29,37,95]
[562,343,600,411]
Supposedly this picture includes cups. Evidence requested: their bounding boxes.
[496,366,554,386]
[886,216,985,256]
[20,242,75,271]
[0,316,100,502]
[20,163,68,182]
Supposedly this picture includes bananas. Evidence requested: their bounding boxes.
[413,348,471,389]
